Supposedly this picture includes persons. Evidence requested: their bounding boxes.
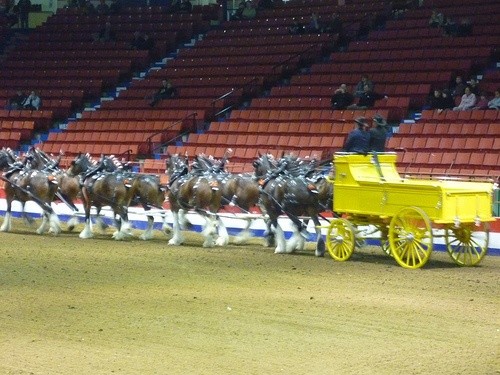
[100,21,115,41]
[68,0,121,15]
[343,114,387,153]
[235,1,256,20]
[430,7,462,37]
[0,0,31,32]
[129,32,152,50]
[333,73,376,109]
[427,74,500,114]
[148,79,176,107]
[9,88,42,111]
[290,12,341,34]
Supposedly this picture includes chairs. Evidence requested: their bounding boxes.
[0,0,500,222]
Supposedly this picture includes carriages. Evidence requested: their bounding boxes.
[0,144,497,271]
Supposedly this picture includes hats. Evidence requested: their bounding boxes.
[354,117,369,126]
[372,115,386,125]
[441,89,448,94]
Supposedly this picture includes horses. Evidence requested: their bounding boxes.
[0,145,333,257]
